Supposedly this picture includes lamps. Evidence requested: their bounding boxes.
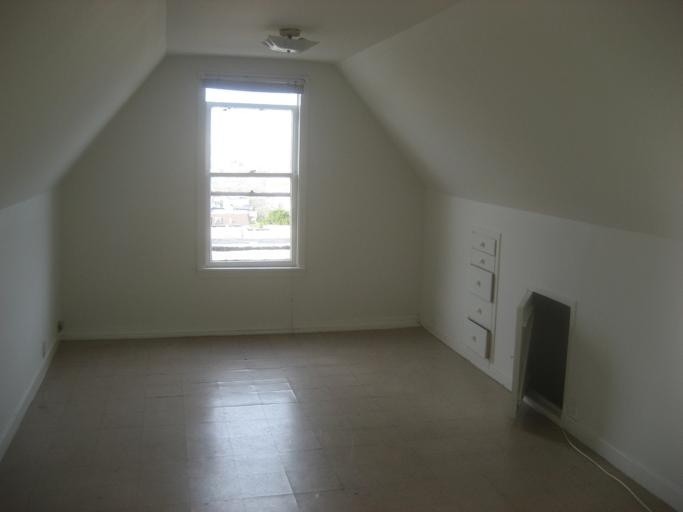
[260,28,320,54]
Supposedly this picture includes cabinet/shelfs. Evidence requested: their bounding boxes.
[466,228,500,365]
